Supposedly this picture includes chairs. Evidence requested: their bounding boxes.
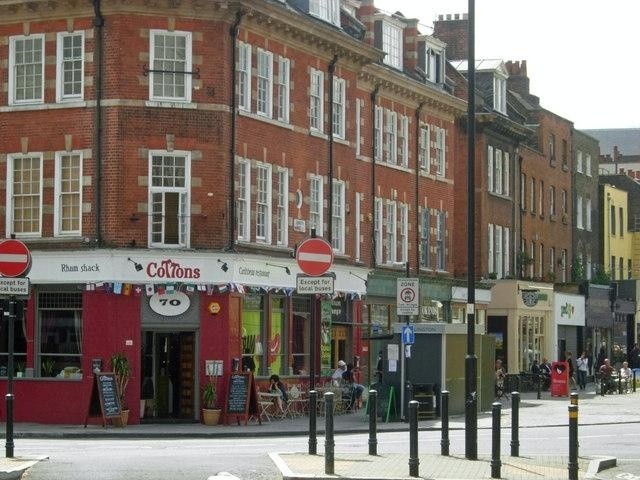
[249,382,370,425]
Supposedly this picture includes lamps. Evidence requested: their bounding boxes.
[127,258,143,271]
[217,259,228,272]
[349,272,368,287]
[266,262,290,276]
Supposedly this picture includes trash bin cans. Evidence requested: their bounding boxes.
[413,380,438,421]
[551,362,569,396]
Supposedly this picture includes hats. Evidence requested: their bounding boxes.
[337,360,346,367]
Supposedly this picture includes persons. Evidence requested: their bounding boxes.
[540,357,552,391]
[566,350,577,390]
[266,374,287,415]
[576,351,589,389]
[615,360,634,394]
[342,363,364,414]
[531,360,542,383]
[331,359,347,387]
[599,358,616,395]
[495,359,505,379]
[376,350,382,383]
[631,342,640,368]
[596,346,606,371]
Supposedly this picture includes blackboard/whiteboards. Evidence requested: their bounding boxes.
[223,372,252,416]
[97,373,123,418]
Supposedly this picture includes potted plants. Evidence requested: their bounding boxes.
[201,361,222,425]
[16,361,26,377]
[110,352,133,427]
